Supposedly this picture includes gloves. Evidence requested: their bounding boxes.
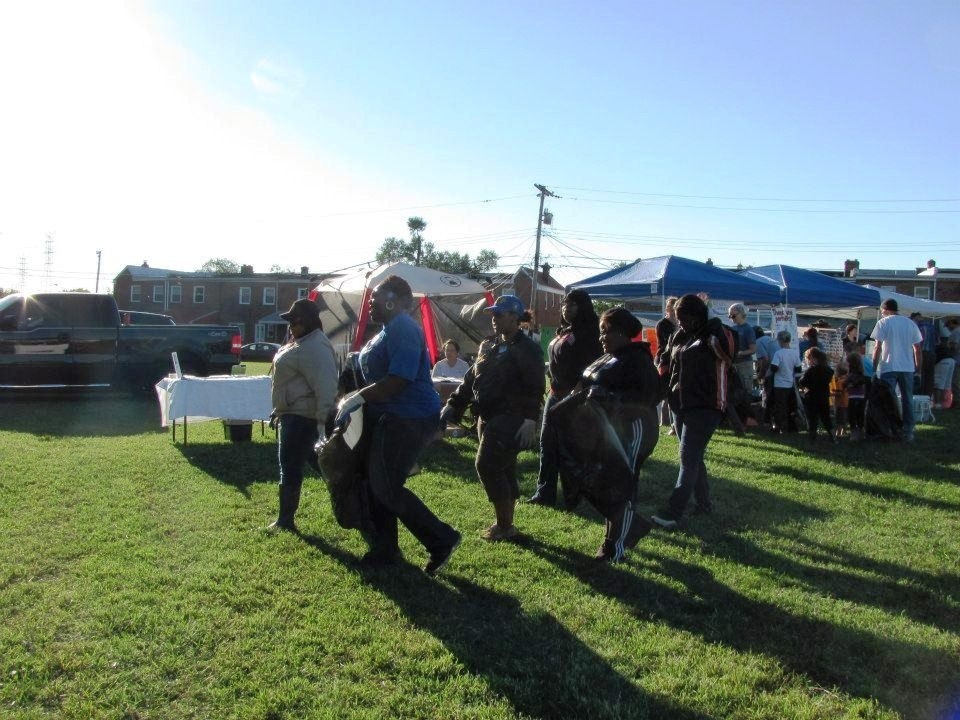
[335,394,366,426]
[514,417,538,450]
[439,403,457,430]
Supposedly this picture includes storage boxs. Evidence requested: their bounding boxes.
[445,424,465,438]
[222,419,254,441]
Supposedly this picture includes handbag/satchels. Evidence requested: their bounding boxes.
[317,428,369,531]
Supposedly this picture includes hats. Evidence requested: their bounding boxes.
[482,295,525,317]
[278,299,320,321]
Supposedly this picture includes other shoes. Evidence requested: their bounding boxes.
[475,522,521,542]
[650,510,678,527]
[261,520,298,533]
[625,523,652,551]
[350,551,402,571]
[560,499,582,515]
[684,506,714,519]
[523,496,556,508]
[590,548,623,565]
[424,530,462,573]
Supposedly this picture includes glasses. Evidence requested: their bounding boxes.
[728,311,740,319]
[288,319,301,327]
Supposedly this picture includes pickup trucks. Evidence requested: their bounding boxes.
[0,292,246,402]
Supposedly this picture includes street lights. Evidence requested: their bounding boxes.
[96,250,101,293]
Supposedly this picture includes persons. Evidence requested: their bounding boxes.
[262,274,960,579]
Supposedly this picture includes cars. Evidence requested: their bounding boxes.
[240,343,282,361]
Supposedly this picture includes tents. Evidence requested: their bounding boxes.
[304,256,960,356]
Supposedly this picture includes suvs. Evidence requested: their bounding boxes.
[118,310,176,325]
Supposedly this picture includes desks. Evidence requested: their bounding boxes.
[431,376,476,402]
[154,373,281,447]
